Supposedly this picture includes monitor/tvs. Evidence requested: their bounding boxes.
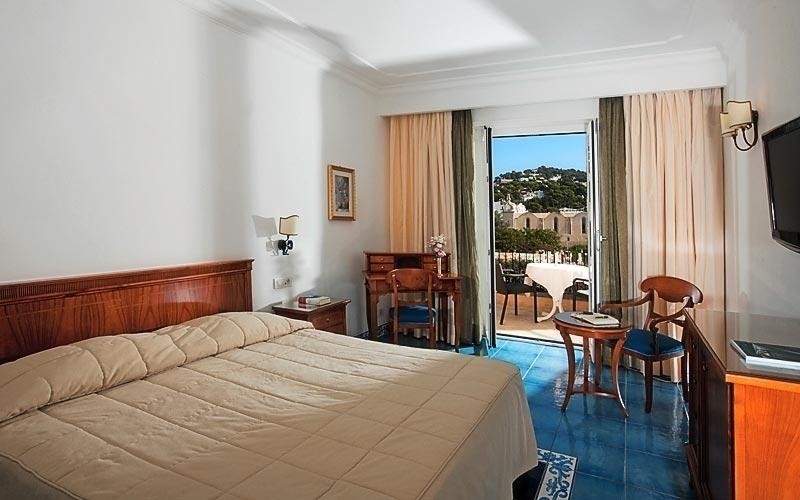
[761,116,800,255]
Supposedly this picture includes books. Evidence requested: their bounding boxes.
[298,292,331,307]
[571,309,621,326]
[729,337,800,370]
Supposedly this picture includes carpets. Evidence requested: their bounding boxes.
[513,445,579,500]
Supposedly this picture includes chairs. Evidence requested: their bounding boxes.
[594,276,703,414]
[496,263,539,325]
[385,267,438,349]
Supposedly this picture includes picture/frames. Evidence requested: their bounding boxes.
[327,164,357,222]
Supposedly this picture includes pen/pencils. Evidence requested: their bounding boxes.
[596,316,608,318]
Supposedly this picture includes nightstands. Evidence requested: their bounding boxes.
[271,297,351,334]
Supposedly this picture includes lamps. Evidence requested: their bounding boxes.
[719,99,759,152]
[278,214,301,256]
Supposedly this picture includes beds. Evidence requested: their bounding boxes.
[0,255,521,500]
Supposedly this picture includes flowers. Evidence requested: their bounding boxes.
[426,232,449,258]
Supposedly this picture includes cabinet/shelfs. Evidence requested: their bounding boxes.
[682,306,800,499]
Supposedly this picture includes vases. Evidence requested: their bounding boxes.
[436,257,442,276]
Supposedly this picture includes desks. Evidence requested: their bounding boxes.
[554,312,633,420]
[527,264,589,322]
[362,251,464,353]
